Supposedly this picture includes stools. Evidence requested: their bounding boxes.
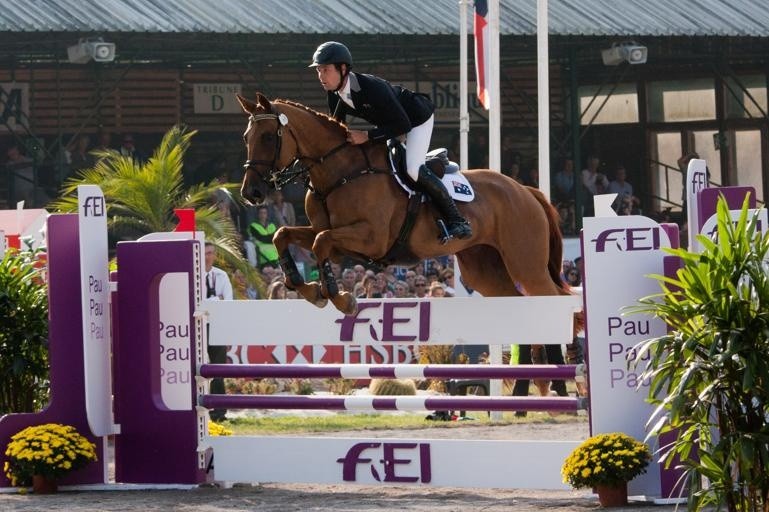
[449,378,490,418]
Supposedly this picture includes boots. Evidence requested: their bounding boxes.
[415,164,473,240]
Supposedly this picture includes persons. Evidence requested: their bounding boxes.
[202,152,687,419]
[2,130,156,271]
[308,40,474,239]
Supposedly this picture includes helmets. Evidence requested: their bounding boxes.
[308,41,352,67]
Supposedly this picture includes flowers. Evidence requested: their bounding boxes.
[4,423,98,495]
[561,432,654,489]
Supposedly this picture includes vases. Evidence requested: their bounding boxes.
[32,472,57,494]
[597,482,627,506]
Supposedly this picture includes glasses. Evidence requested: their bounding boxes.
[415,283,426,287]
[443,275,452,281]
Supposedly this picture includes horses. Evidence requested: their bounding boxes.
[235,91,578,418]
